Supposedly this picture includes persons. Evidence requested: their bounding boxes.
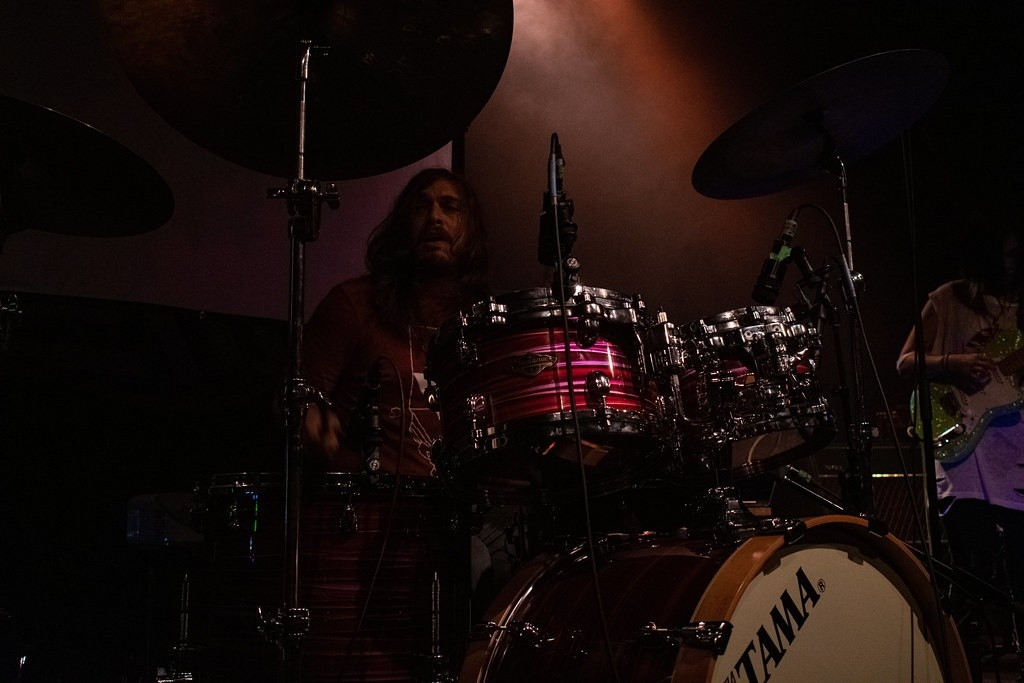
[293,169,490,596]
[896,217,1024,660]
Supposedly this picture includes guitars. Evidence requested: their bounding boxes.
[909,325,1024,463]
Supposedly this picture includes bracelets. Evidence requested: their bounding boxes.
[941,351,952,370]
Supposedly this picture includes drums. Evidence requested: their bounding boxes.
[124,470,270,556]
[664,306,838,490]
[230,471,466,683]
[454,513,976,683]
[421,282,685,505]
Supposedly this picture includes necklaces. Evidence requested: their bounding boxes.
[994,293,1015,321]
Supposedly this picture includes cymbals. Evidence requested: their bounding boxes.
[90,0,515,183]
[690,47,951,201]
[0,94,177,241]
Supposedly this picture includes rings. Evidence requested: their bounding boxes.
[977,372,980,376]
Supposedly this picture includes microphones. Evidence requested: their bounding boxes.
[539,138,574,267]
[752,206,802,304]
[287,175,320,241]
